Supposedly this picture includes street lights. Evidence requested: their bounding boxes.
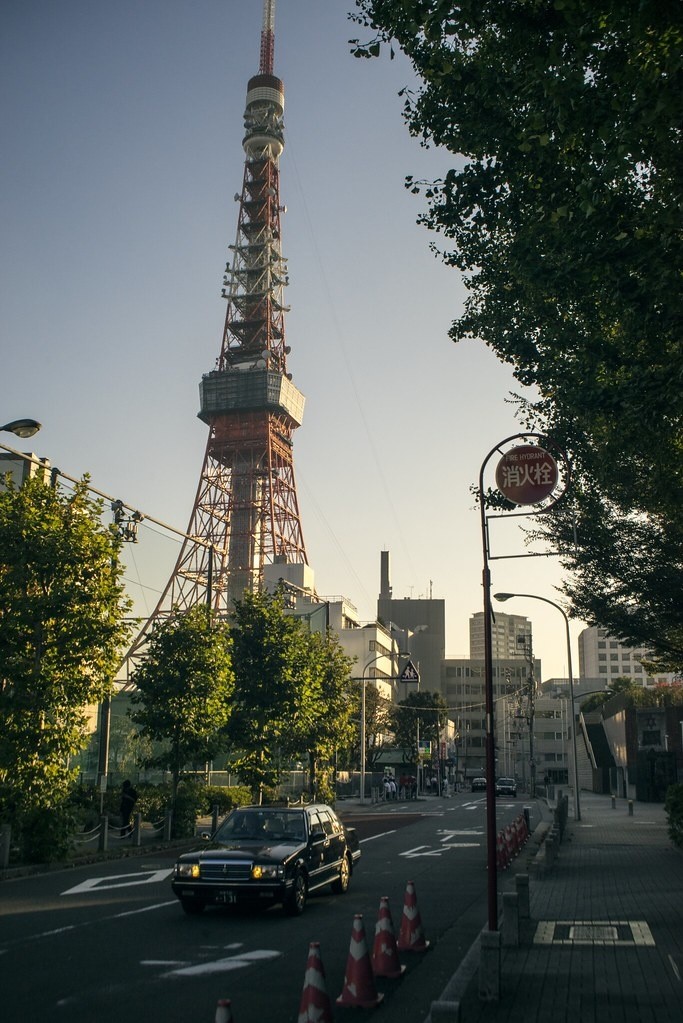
[515,715,536,800]
[493,592,615,823]
[359,651,412,804]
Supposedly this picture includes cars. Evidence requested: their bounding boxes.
[471,778,487,793]
[172,801,361,920]
[495,777,517,796]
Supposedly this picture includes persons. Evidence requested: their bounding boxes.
[544,775,548,788]
[241,813,265,834]
[425,774,448,796]
[382,772,416,800]
[120,780,137,838]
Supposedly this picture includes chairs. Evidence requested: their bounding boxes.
[265,818,306,834]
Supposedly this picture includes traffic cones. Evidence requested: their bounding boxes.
[215,998,233,1023]
[372,896,406,979]
[396,881,430,953]
[497,816,530,869]
[335,914,385,1009]
[298,942,333,1023]
